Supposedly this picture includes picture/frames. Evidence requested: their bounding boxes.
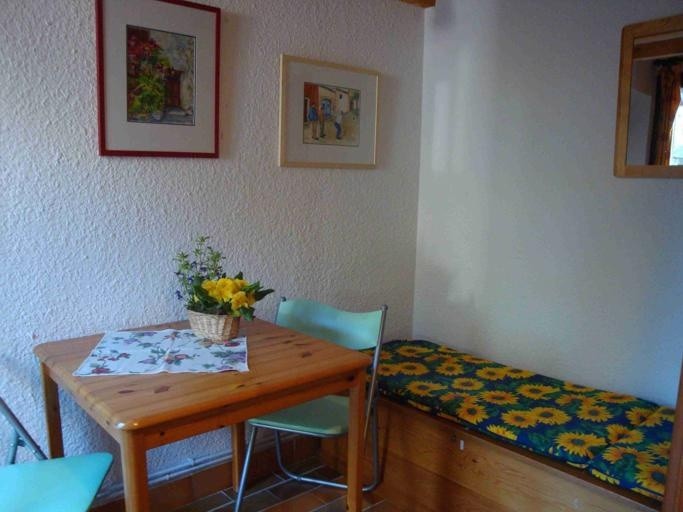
[96,0,220,158]
[279,54,381,169]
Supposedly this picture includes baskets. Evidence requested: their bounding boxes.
[186,307,241,342]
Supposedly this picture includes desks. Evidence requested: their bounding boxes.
[32,312,372,512]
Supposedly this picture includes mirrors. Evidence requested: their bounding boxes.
[613,12,683,179]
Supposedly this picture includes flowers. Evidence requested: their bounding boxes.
[170,234,274,322]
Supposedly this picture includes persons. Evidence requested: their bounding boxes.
[308,101,320,141]
[333,111,345,140]
[318,107,327,138]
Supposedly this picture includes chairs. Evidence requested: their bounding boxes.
[235,294,389,512]
[0,395,114,512]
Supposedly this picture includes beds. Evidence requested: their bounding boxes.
[319,338,681,511]
